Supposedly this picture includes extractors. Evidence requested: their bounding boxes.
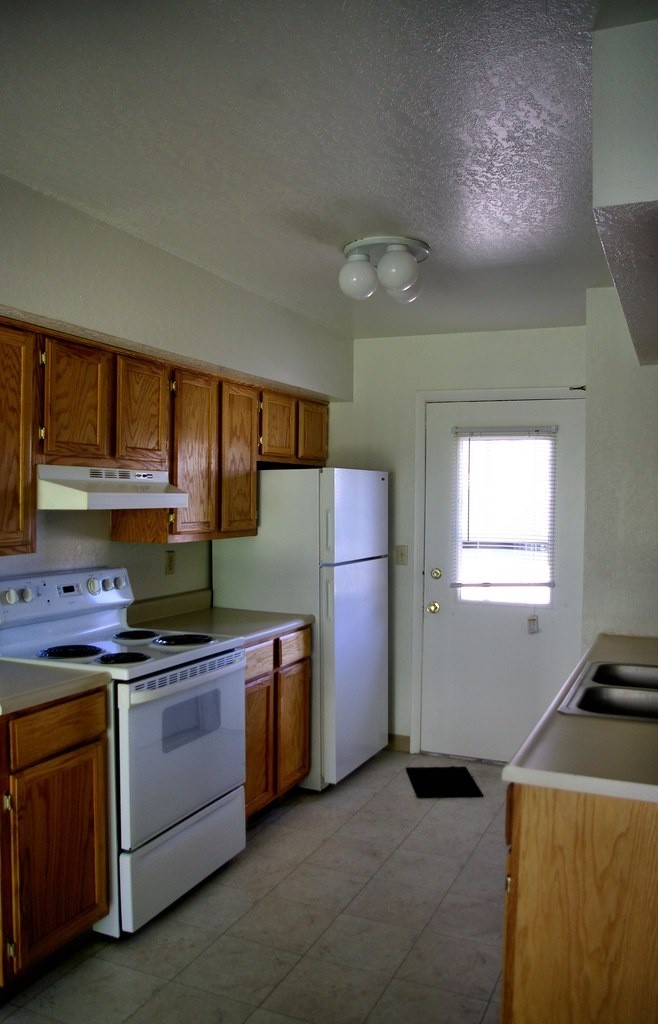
[34,464,189,511]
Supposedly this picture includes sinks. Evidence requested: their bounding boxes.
[577,686,658,721]
[593,664,658,688]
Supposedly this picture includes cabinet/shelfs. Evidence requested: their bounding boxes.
[257,386,329,471]
[240,624,312,820]
[0,316,41,555]
[109,364,261,545]
[40,326,172,472]
[0,685,111,988]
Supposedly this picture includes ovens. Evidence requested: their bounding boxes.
[91,649,247,939]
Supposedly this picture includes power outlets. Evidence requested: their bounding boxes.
[164,550,176,575]
[395,545,408,566]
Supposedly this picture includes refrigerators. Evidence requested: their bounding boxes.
[211,468,389,792]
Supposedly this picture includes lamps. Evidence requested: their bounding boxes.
[338,236,430,304]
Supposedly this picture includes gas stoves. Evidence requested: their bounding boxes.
[0,564,245,682]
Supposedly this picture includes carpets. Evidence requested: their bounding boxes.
[405,765,484,798]
